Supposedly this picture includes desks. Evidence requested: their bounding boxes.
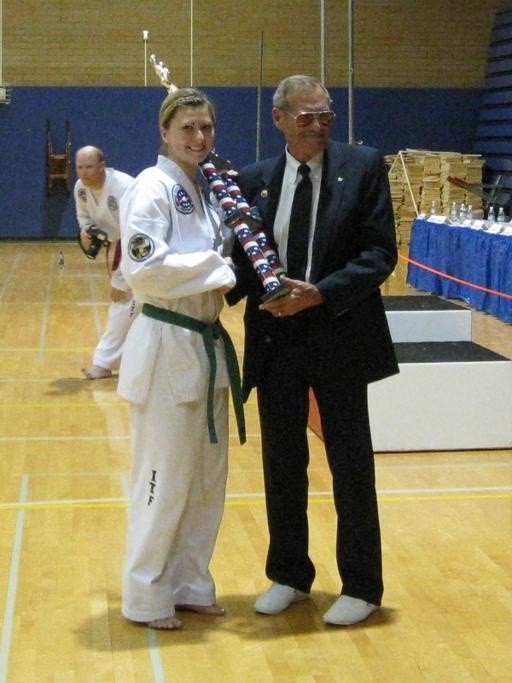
[407,218,511,322]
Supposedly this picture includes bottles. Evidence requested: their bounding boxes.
[58,251,64,271]
[431,200,505,225]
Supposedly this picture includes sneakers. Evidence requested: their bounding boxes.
[322,595,377,626]
[254,581,304,614]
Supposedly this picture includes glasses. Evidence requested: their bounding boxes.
[284,109,334,130]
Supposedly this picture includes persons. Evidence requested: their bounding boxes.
[220,76,403,626]
[73,146,136,380]
[124,87,237,629]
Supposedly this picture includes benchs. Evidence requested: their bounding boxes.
[382,294,471,341]
[308,341,512,451]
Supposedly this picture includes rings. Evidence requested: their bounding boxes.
[279,312,283,318]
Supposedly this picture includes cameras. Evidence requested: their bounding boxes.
[86,224,108,259]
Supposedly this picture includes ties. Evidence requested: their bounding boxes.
[286,165,313,282]
